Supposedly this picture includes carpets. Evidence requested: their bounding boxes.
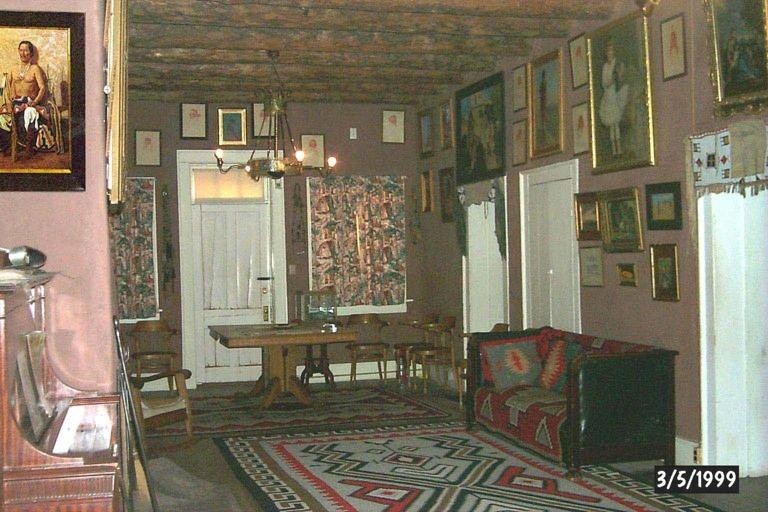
[213,420,726,512]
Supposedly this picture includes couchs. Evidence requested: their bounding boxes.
[465,323,678,479]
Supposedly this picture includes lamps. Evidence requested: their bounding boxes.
[214,47,337,181]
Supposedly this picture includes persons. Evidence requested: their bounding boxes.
[598,40,629,155]
[0,40,46,159]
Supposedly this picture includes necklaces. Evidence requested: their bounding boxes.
[17,63,31,80]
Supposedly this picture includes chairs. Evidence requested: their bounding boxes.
[127,319,181,396]
[122,343,194,443]
[344,314,462,409]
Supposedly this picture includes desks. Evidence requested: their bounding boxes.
[208,323,357,411]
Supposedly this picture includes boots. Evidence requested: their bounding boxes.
[610,137,625,159]
[22,127,35,160]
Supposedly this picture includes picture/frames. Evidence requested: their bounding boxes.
[456,72,507,185]
[649,243,679,302]
[660,13,686,81]
[645,183,683,231]
[218,108,248,147]
[704,0,768,120]
[253,102,278,139]
[587,7,656,169]
[512,119,527,166]
[181,103,209,141]
[439,169,459,223]
[382,109,406,143]
[417,109,436,158]
[0,12,86,193]
[512,63,528,112]
[531,50,564,160]
[440,101,454,149]
[573,192,601,240]
[567,33,588,90]
[599,187,644,256]
[134,129,160,167]
[421,171,434,212]
[300,132,326,168]
[580,246,604,288]
